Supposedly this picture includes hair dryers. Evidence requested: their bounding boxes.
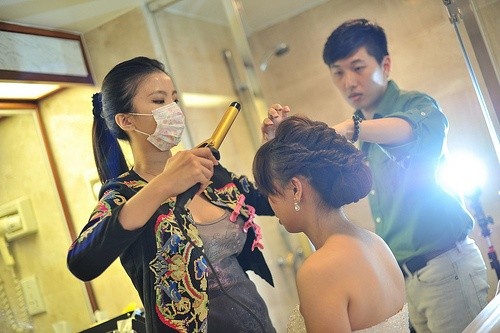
[0,214,23,267]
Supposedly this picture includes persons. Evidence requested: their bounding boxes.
[67,56,290,333]
[322,19,490,333]
[253,115,411,333]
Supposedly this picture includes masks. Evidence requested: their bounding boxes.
[129,102,184,151]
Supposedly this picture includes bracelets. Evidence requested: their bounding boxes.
[351,115,362,143]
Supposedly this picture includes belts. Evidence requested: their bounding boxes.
[399,234,466,278]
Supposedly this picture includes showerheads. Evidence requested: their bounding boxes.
[259,42,289,72]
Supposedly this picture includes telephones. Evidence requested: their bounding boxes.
[0,237,16,267]
